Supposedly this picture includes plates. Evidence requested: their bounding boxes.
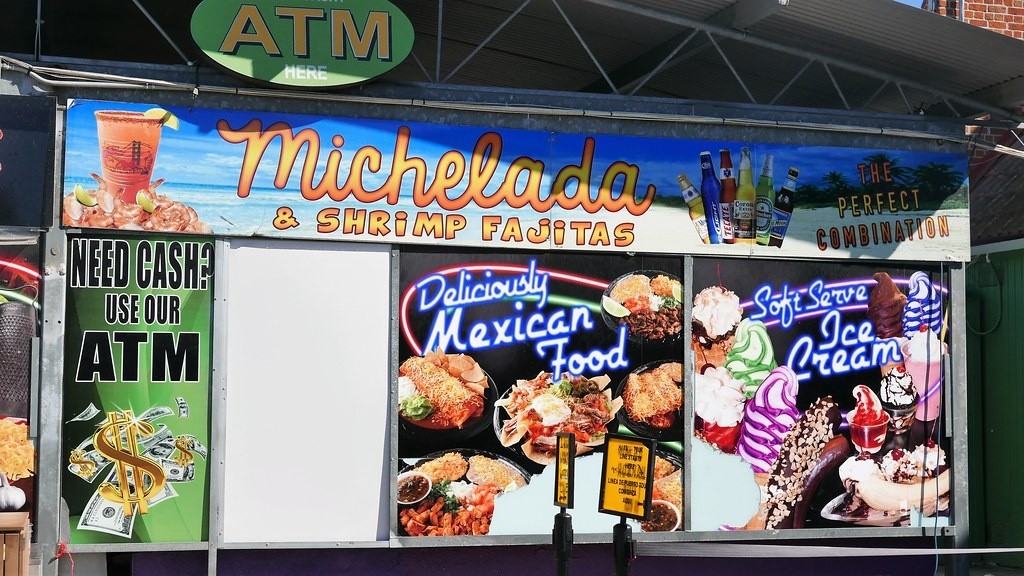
[574,444,684,532]
[600,270,684,344]
[493,383,619,464]
[397,471,432,507]
[399,367,499,441]
[396,449,531,539]
[636,499,680,532]
[617,361,682,439]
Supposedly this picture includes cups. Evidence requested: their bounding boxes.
[845,410,889,453]
[93,109,162,205]
[878,338,907,375]
[902,339,948,421]
[876,390,921,435]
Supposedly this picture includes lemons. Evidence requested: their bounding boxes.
[602,294,632,318]
[136,190,157,213]
[144,108,178,130]
[75,183,98,207]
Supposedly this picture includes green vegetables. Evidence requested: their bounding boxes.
[421,477,461,516]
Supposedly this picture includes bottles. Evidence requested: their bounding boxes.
[678,173,710,244]
[735,147,756,244]
[700,151,721,244]
[755,154,775,245]
[720,148,736,244]
[770,167,800,248]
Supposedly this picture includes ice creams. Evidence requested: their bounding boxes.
[690,270,946,532]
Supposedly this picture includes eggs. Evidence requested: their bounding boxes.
[532,394,572,426]
